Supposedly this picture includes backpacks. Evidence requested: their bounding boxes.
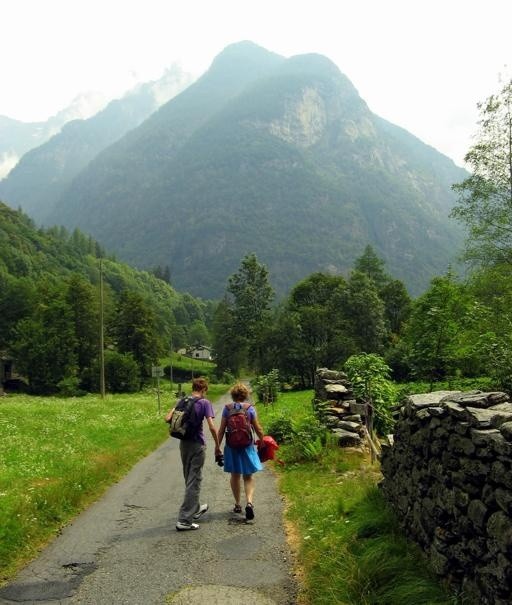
[225,403,254,448]
[169,397,199,440]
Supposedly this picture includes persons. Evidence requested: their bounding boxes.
[215,382,266,525]
[164,377,224,531]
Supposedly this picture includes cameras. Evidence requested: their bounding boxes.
[216,455,225,466]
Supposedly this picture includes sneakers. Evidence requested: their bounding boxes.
[193,504,208,520]
[176,522,199,531]
[234,504,242,512]
[245,503,254,520]
